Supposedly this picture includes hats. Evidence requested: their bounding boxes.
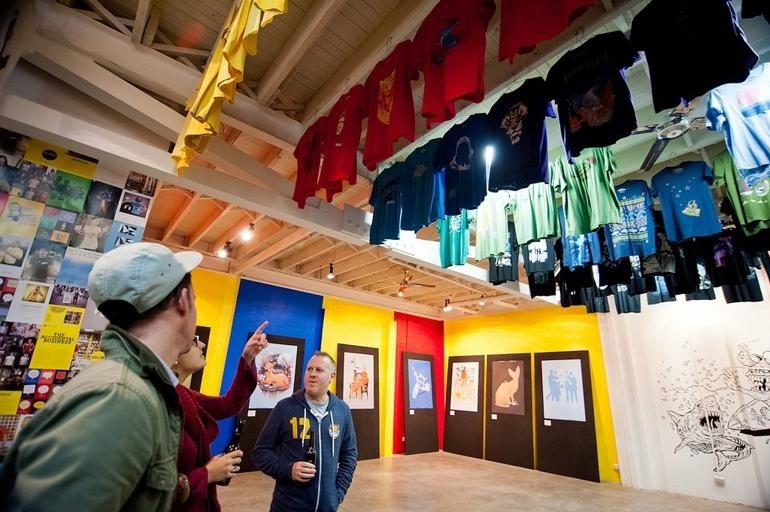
[89,242,203,314]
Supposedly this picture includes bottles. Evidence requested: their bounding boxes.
[301,428,317,465]
[213,417,249,487]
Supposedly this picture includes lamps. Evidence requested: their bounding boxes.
[218,240,232,258]
[478,296,486,306]
[442,299,453,312]
[396,287,405,297]
[327,263,335,280]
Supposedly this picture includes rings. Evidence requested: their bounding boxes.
[232,466,237,472]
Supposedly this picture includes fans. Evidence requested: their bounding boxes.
[375,267,437,297]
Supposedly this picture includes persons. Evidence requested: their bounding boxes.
[0,152,158,252]
[53,285,89,305]
[249,351,359,512]
[25,285,45,302]
[542,369,581,403]
[1,321,40,376]
[170,320,269,509]
[1,240,205,511]
[24,247,58,283]
